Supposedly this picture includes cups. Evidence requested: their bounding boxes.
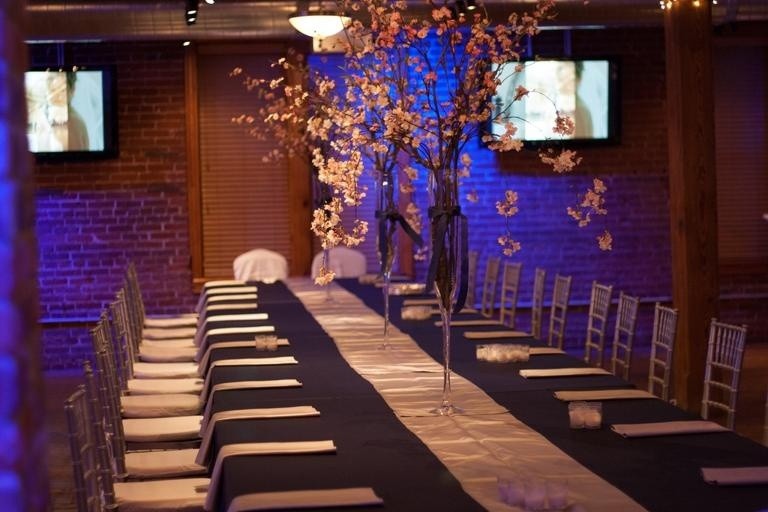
[388,282,428,298]
[474,342,532,365]
[266,334,278,351]
[491,465,570,511]
[569,400,604,431]
[255,335,266,352]
[399,303,436,322]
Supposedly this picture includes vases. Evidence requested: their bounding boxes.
[374,170,396,352]
[426,169,466,417]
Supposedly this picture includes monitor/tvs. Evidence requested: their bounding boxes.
[24,64,119,164]
[477,55,621,148]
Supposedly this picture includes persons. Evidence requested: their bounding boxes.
[38,71,89,153]
[548,60,592,140]
